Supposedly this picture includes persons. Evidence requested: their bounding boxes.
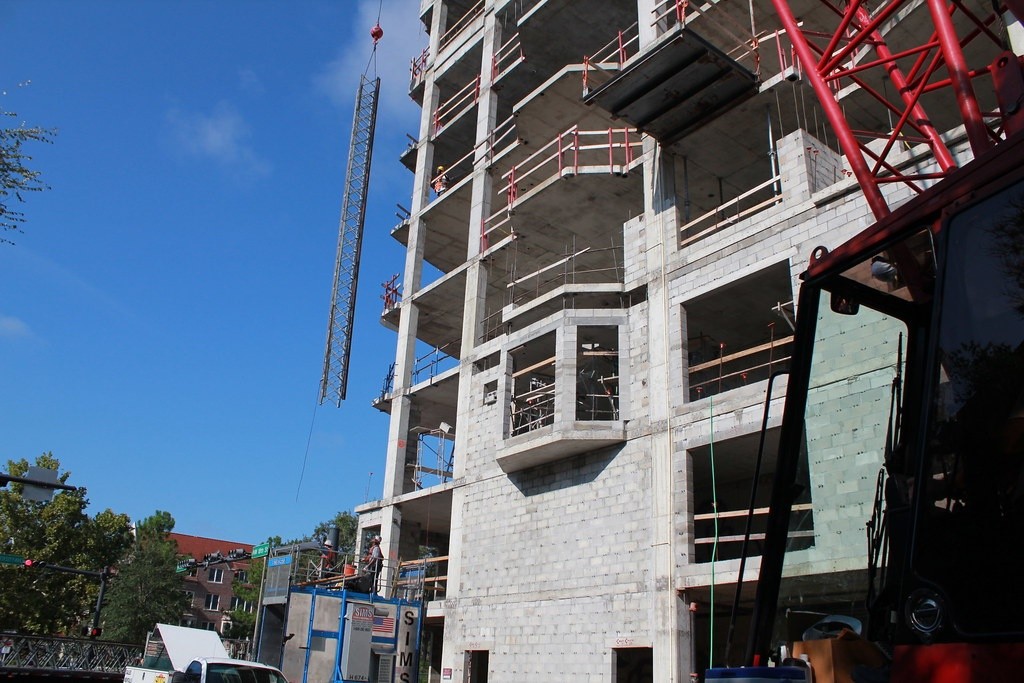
[362,534,383,595]
[435,166,449,197]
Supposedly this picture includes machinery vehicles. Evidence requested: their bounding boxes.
[702,2,1024,682]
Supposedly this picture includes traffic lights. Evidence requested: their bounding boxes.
[25,558,47,569]
[203,551,222,563]
[228,549,246,558]
[87,627,102,638]
[178,559,197,570]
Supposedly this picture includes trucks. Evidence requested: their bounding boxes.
[122,623,288,683]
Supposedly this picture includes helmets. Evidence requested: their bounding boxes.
[324,540,334,547]
[375,535,381,543]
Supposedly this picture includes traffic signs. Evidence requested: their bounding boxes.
[251,542,269,558]
[0,553,24,564]
[176,564,187,573]
